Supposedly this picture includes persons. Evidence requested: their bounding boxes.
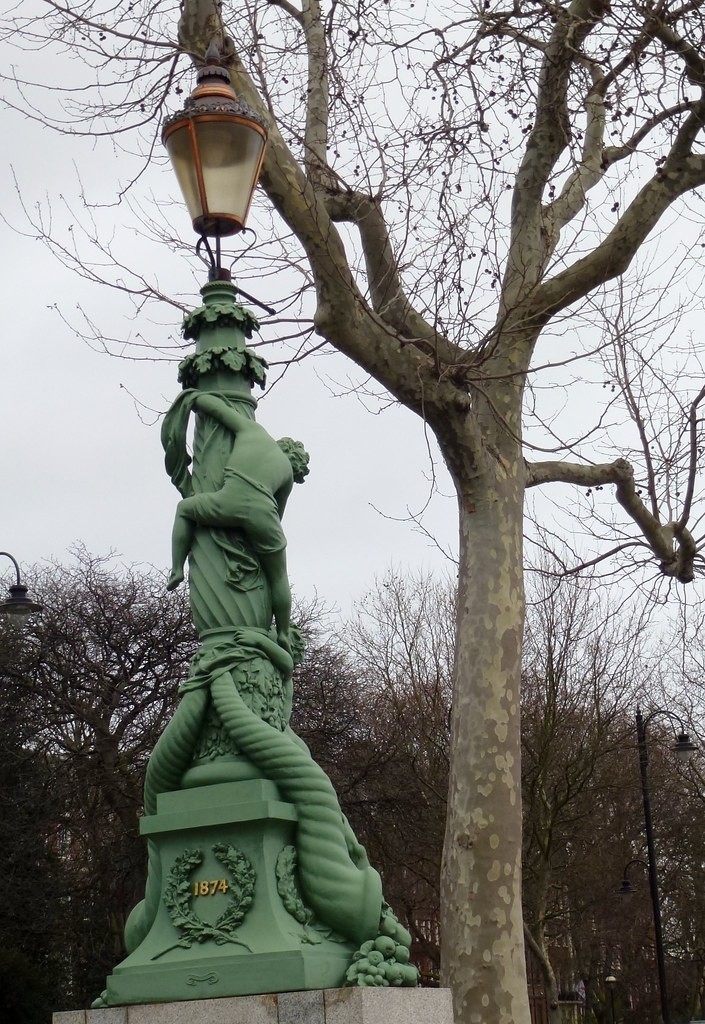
[166,391,311,650]
[233,620,373,870]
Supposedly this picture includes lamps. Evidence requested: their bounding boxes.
[159,62,270,277]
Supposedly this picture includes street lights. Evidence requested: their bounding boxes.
[604,969,616,1024]
[612,696,700,1024]
[90,31,425,1017]
